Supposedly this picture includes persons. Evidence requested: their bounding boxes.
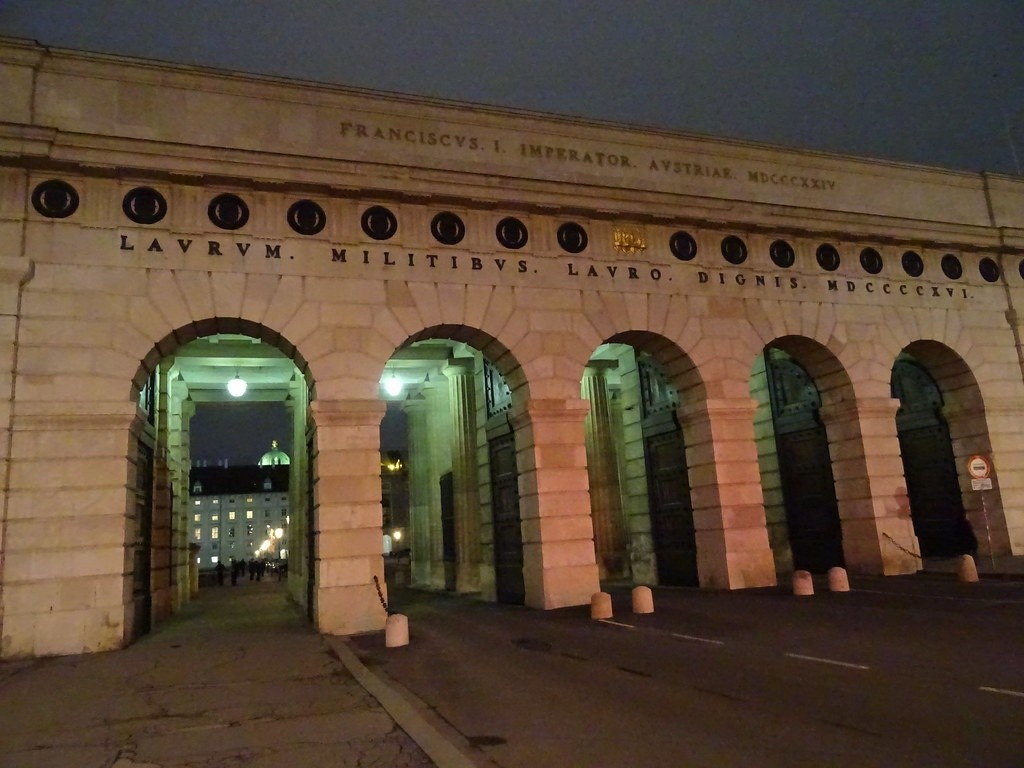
[240,558,247,578]
[249,558,265,582]
[215,560,227,586]
[230,559,240,587]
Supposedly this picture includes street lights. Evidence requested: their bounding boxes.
[264,539,270,574]
[392,531,401,567]
[274,527,284,583]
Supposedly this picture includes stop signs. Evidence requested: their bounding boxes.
[966,453,991,478]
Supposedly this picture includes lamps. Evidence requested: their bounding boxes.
[384,366,404,395]
[227,366,247,397]
